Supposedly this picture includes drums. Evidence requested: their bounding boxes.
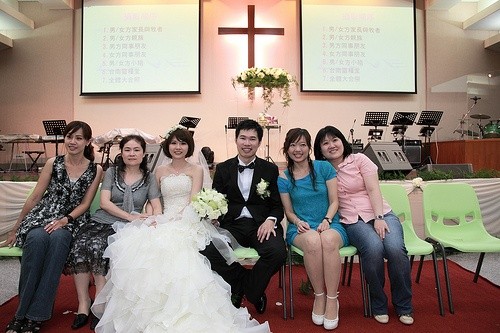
[482,123,500,137]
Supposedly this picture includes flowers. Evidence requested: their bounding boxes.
[256,179,271,200]
[190,188,229,221]
[232,66,299,114]
[163,124,180,140]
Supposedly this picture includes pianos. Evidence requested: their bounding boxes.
[0,133,65,171]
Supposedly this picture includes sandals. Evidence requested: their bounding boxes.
[4,317,43,333]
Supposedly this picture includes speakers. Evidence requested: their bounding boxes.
[347,140,473,179]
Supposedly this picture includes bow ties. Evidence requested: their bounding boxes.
[237,162,255,173]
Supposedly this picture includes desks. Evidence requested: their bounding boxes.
[97,142,161,168]
[0,139,51,173]
[225,125,281,165]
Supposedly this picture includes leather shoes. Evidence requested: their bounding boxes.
[255,292,267,314]
[231,291,244,308]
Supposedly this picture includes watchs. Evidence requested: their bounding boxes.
[65,214,73,224]
[323,216,333,225]
[375,214,385,220]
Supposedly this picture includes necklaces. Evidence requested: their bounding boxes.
[172,162,187,174]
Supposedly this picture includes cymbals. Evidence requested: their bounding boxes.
[470,113,491,119]
[455,129,479,136]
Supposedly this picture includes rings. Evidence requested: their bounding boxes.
[50,221,54,225]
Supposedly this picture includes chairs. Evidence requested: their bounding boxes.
[416,183,500,313]
[234,184,444,317]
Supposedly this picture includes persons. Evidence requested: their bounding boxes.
[201,119,288,314]
[0,121,103,333]
[277,127,348,330]
[90,127,270,333]
[72,135,162,330]
[314,126,415,325]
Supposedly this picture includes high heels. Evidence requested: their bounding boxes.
[70,298,94,328]
[311,292,339,330]
[91,315,101,329]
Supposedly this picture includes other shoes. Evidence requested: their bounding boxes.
[399,315,415,325]
[374,313,389,324]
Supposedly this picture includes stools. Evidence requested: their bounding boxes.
[22,151,45,172]
